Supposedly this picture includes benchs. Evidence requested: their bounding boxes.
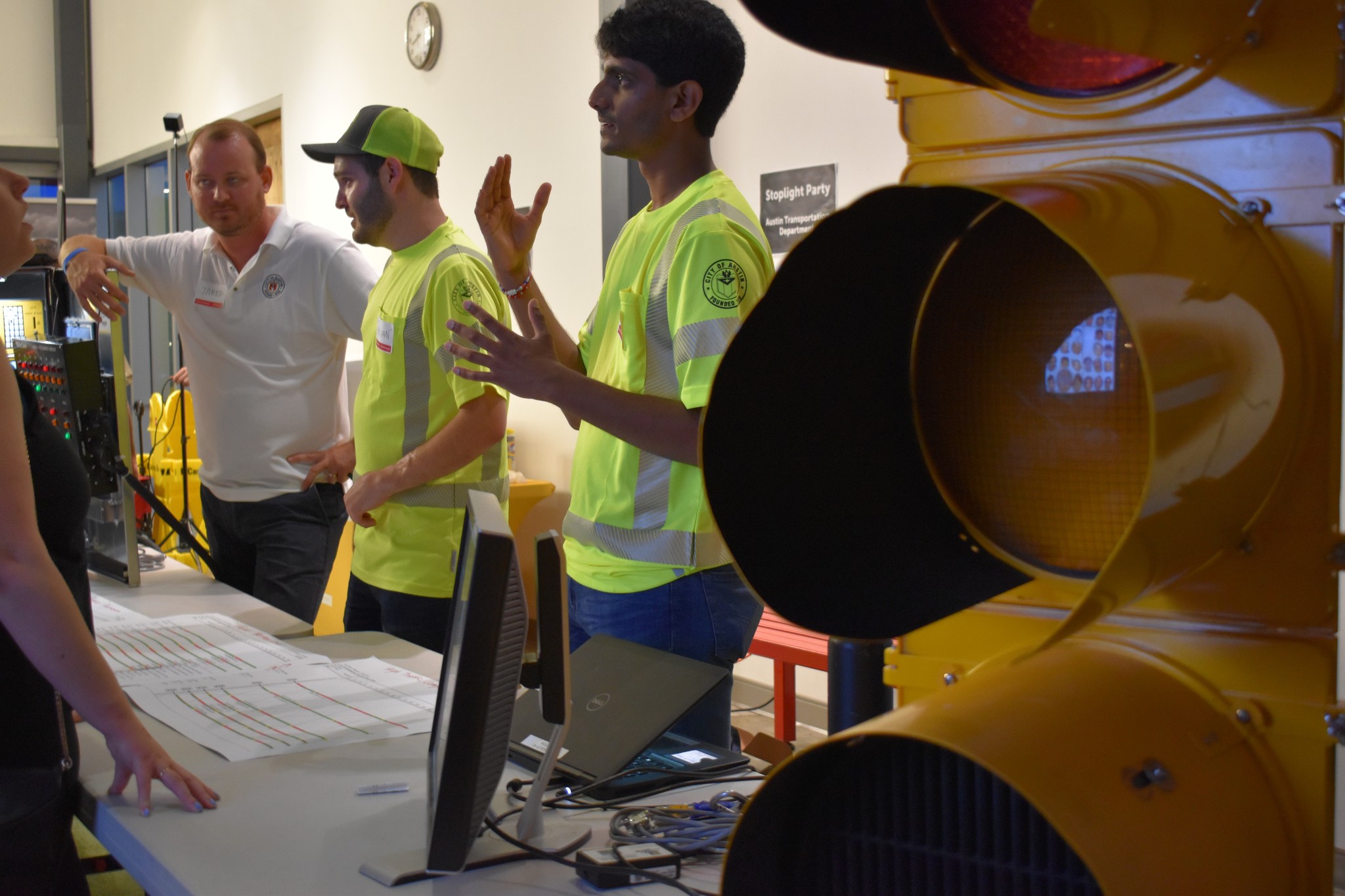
[734,605,828,741]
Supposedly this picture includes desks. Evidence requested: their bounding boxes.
[89,541,313,638]
[67,631,763,896]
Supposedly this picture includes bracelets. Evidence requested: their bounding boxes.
[63,248,88,273]
[501,267,531,298]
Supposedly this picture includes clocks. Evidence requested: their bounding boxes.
[406,2,441,72]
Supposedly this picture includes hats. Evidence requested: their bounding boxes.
[299,105,441,176]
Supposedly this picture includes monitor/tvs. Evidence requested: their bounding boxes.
[1,267,53,369]
[423,489,529,837]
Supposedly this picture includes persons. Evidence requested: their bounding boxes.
[120,350,139,493]
[443,0,777,745]
[169,367,190,386]
[300,105,511,659]
[59,120,382,625]
[1,156,225,895]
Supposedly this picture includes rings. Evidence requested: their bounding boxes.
[158,766,171,780]
[325,473,338,478]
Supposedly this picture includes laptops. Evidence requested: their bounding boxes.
[507,630,751,799]
